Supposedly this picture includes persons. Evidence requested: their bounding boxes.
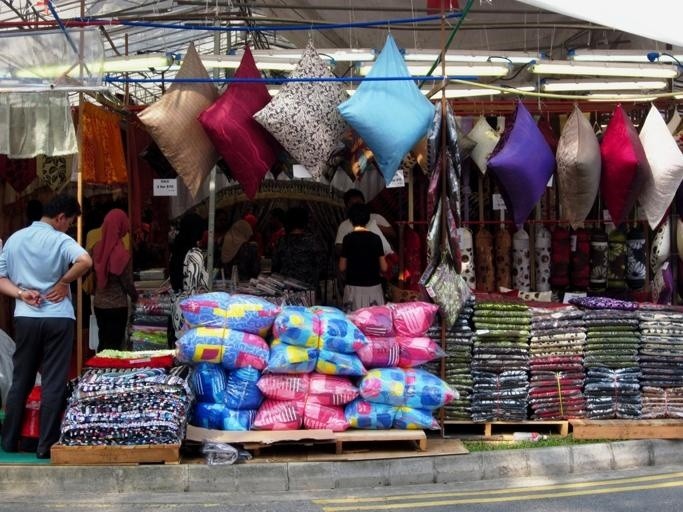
[331,187,422,313]
[0,193,92,458]
[164,208,289,293]
[85,208,153,354]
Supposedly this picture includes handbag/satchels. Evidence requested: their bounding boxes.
[83,271,96,293]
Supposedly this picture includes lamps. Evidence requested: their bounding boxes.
[12,53,173,78]
[267,85,282,96]
[345,45,682,104]
[236,48,376,62]
[184,53,336,71]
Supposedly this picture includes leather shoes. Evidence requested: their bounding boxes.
[34,450,51,458]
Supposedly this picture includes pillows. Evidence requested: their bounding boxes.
[253,39,364,184]
[341,31,437,187]
[142,35,219,206]
[196,36,282,199]
[451,99,683,234]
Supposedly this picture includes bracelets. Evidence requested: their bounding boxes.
[15,288,25,300]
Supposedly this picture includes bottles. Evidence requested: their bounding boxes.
[512,431,547,443]
[230,265,240,295]
